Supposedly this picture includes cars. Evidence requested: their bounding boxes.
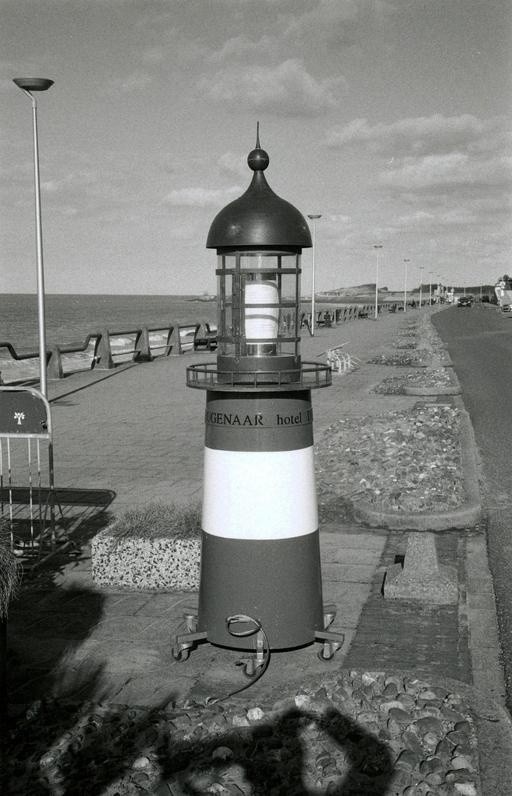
[457,297,471,308]
[467,296,474,303]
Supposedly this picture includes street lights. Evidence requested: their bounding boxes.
[401,258,410,312]
[372,244,383,319]
[416,267,456,308]
[11,78,58,556]
[303,213,323,338]
